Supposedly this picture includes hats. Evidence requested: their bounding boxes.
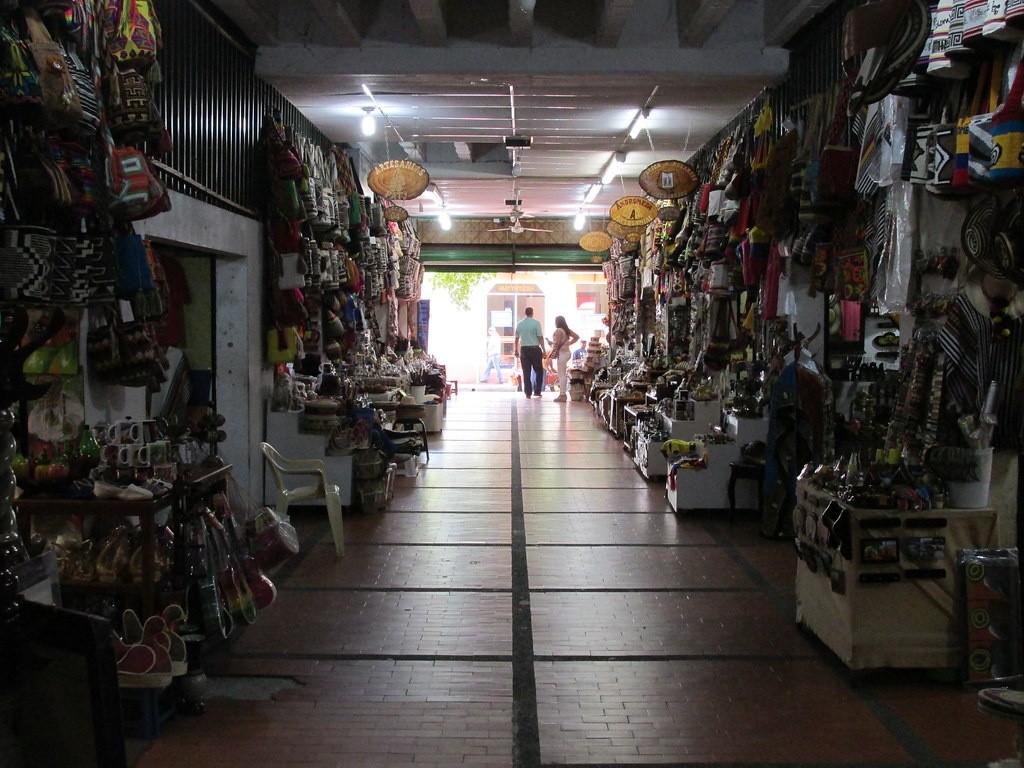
[836,0,931,103]
[580,339,588,343]
[990,192,1024,289]
[964,264,1024,345]
[960,190,1008,280]
[740,437,768,465]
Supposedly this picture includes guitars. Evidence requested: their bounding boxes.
[185,494,278,642]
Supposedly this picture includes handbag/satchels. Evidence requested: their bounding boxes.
[259,104,422,365]
[0,0,171,396]
[899,0,1024,200]
[601,201,681,348]
[548,347,559,359]
[667,75,868,372]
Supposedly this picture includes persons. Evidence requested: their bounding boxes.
[548,316,579,402]
[573,340,588,359]
[481,326,504,385]
[515,307,558,398]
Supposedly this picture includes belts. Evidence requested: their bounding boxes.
[521,346,539,349]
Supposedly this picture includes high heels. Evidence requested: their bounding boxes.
[554,394,567,403]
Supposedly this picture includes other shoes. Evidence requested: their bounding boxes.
[93,480,154,501]
[119,476,173,498]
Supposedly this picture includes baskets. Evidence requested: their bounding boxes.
[569,368,585,401]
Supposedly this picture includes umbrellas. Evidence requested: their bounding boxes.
[580,196,658,252]
[658,207,681,222]
[383,206,408,223]
[367,159,430,200]
[639,160,698,199]
